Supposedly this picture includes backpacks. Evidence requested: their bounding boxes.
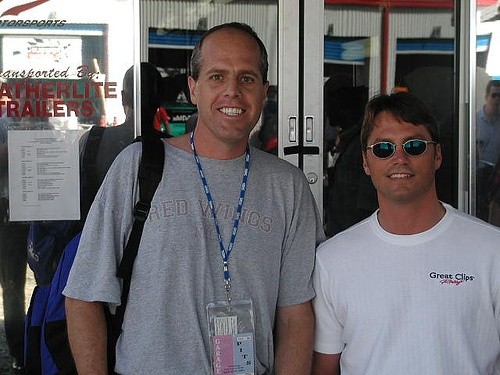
[25,125,106,275]
[24,136,164,375]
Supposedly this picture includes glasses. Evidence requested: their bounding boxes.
[491,92,500,97]
[366,139,437,159]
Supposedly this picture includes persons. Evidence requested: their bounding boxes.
[312,91,500,375]
[62,21,326,375]
[0,62,278,375]
[476,80,500,227]
[323,73,379,237]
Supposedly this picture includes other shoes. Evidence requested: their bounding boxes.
[11,358,23,373]
